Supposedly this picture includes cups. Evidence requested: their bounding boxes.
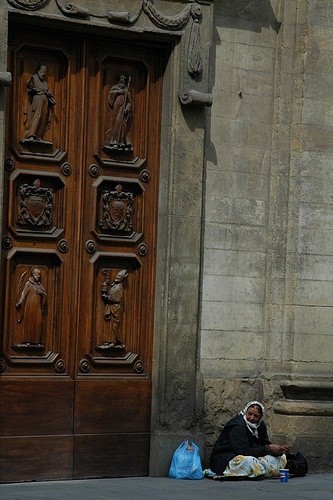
[279,469,289,484]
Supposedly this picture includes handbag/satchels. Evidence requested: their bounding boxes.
[168,440,203,480]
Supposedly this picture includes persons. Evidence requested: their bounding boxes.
[15,267,48,348]
[18,66,57,143]
[99,268,129,341]
[207,400,289,481]
[107,74,134,148]
